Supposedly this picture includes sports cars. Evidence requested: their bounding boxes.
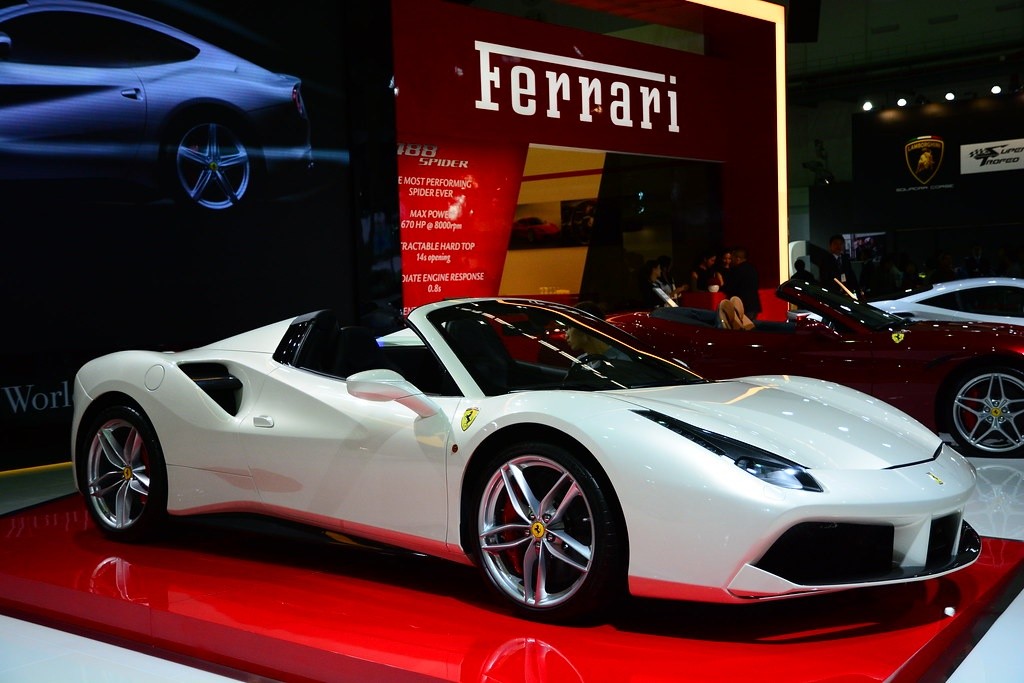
[512,217,560,243]
[0,0,353,215]
[486,278,1024,457]
[70,297,983,623]
[787,277,1024,328]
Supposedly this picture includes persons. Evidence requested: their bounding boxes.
[643,247,761,321]
[562,302,634,383]
[855,238,880,260]
[791,259,816,311]
[811,234,857,292]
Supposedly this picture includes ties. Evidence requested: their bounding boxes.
[837,256,841,268]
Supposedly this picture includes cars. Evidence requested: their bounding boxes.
[562,205,596,245]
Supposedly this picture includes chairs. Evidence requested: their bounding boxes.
[717,297,755,330]
[448,311,524,391]
[321,325,387,379]
